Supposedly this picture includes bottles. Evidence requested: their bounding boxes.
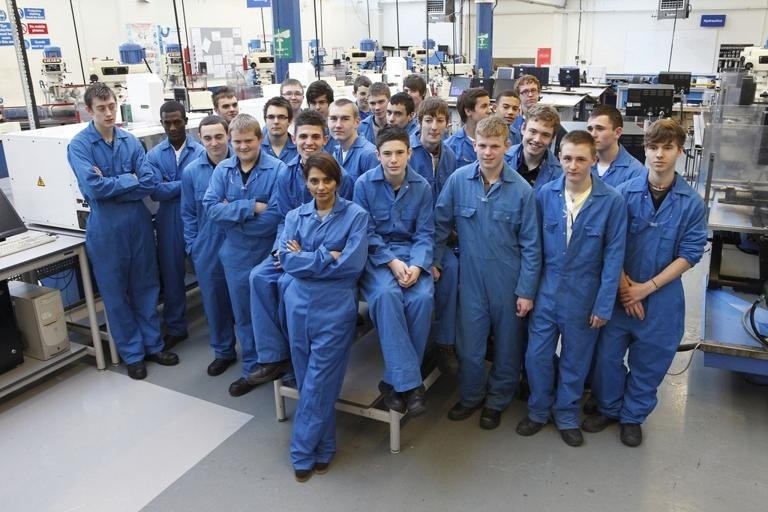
[717,50,740,73]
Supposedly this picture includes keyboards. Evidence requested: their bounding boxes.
[0,231,56,260]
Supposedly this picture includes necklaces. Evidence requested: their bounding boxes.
[649,182,669,190]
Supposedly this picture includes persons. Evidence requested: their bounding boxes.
[404,97,458,381]
[246,108,351,385]
[517,131,628,446]
[202,113,287,396]
[276,152,369,479]
[326,98,380,181]
[145,101,206,349]
[434,116,542,430]
[582,119,707,446]
[261,76,569,172]
[352,124,435,417]
[501,102,563,401]
[68,80,179,380]
[581,105,643,412]
[212,86,240,121]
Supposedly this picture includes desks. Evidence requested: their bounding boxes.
[0,231,107,405]
[670,101,705,113]
[274,291,441,455]
[446,82,611,121]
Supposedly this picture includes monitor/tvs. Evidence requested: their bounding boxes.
[526,66,549,89]
[492,78,516,99]
[625,88,673,120]
[1,186,27,239]
[448,77,472,98]
[658,72,692,94]
[558,67,581,92]
[495,66,515,79]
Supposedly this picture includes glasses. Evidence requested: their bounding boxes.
[520,88,539,96]
[265,114,289,120]
[282,90,304,98]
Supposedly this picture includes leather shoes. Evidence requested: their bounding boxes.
[405,384,426,416]
[316,463,328,473]
[437,345,642,447]
[295,469,311,481]
[379,380,406,413]
[127,335,295,397]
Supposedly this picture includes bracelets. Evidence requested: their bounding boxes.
[652,280,657,288]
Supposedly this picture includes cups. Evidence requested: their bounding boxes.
[644,120,650,132]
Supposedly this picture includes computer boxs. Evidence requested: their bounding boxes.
[9,280,71,359]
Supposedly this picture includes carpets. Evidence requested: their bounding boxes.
[0,359,255,511]
